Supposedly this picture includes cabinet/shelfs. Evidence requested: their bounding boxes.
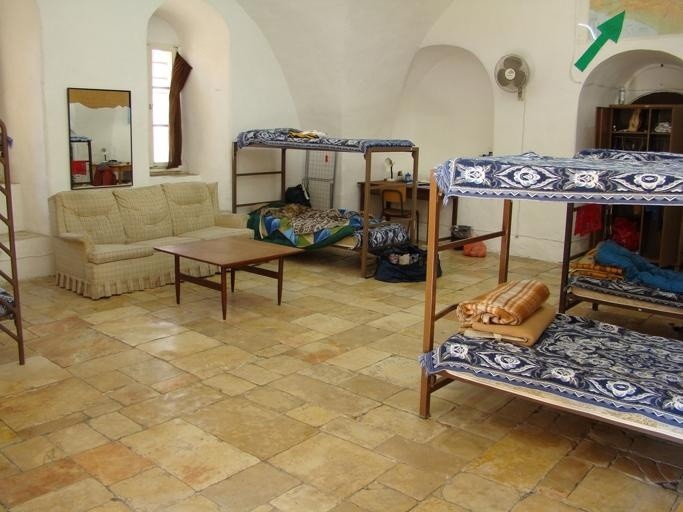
[597,104,683,272]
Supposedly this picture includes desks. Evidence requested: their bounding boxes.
[357,176,458,240]
[92,159,132,182]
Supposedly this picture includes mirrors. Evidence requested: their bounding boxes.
[68,88,134,190]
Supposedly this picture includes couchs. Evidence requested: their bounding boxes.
[47,181,254,301]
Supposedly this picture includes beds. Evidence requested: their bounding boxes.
[420,150,683,444]
[559,202,683,316]
[231,127,419,278]
[73,129,93,181]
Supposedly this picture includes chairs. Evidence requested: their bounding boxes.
[378,183,419,244]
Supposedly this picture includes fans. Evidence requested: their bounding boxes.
[494,54,530,101]
